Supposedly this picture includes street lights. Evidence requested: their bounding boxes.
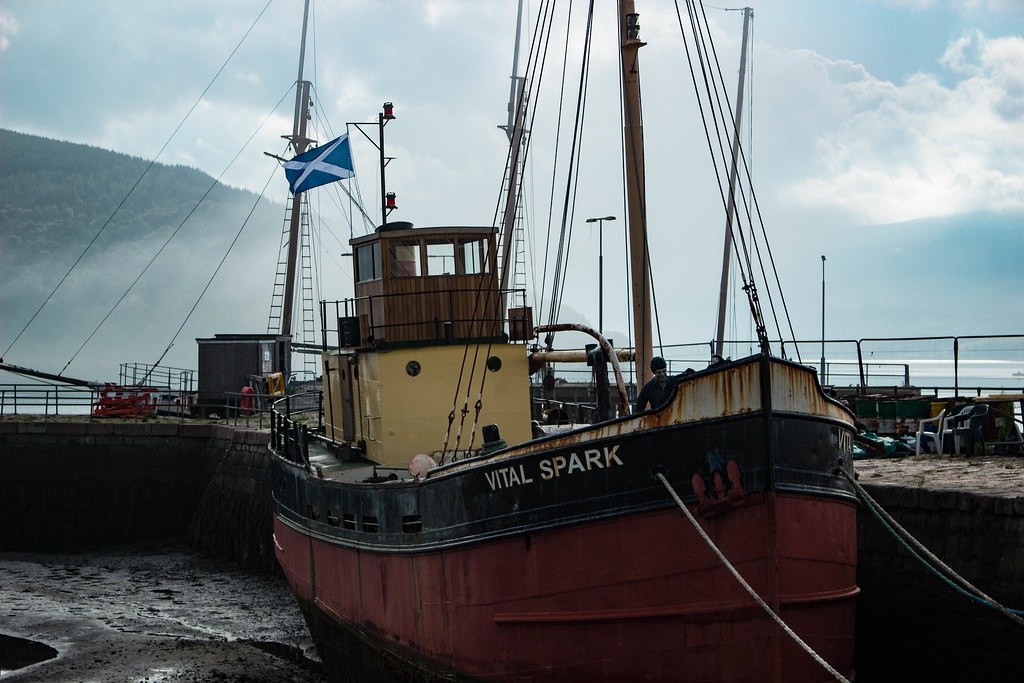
[819,255,828,385]
[585,215,616,341]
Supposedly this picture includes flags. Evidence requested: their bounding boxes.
[283,131,353,195]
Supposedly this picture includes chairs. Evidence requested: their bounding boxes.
[942,405,975,454]
[915,408,946,456]
[951,404,990,458]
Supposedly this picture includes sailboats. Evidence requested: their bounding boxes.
[0,1,871,682]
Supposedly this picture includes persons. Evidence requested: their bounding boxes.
[635,357,676,414]
